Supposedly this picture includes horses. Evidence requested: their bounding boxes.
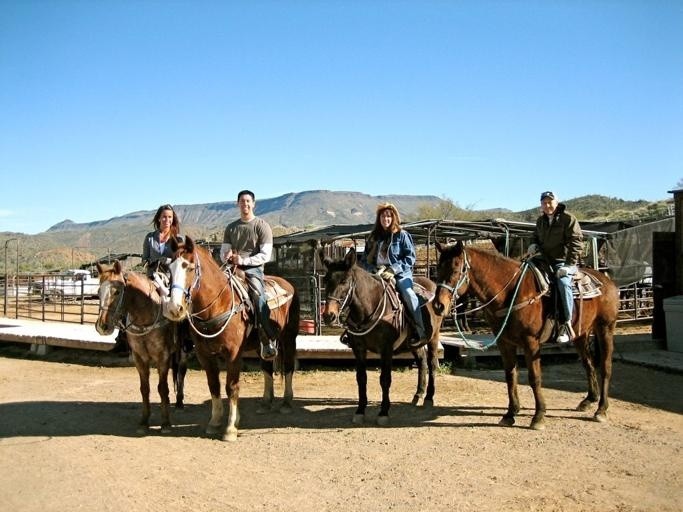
[165,233,302,444]
[318,246,445,428]
[429,237,622,431]
[94,259,189,436]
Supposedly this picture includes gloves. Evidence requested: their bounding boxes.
[560,266,569,276]
[382,268,395,280]
[527,244,536,254]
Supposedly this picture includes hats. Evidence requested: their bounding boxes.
[541,191,554,200]
[375,202,401,224]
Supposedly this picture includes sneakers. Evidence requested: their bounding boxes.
[410,338,425,347]
[263,340,275,359]
[557,327,570,344]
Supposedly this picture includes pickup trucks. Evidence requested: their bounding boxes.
[32,269,100,302]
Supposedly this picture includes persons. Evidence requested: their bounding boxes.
[218,191,279,361]
[528,192,584,344]
[107,204,193,357]
[360,204,427,349]
